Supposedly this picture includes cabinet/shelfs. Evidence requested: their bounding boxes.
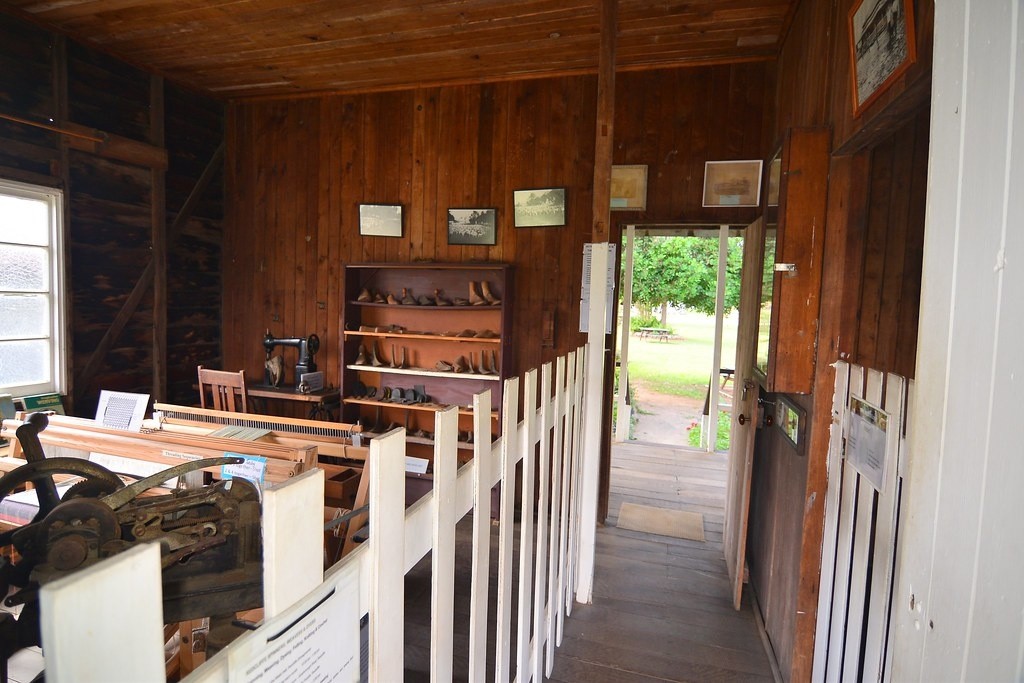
[339,263,516,522]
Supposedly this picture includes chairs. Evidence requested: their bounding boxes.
[196,364,251,414]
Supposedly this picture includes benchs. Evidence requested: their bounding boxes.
[636,332,670,339]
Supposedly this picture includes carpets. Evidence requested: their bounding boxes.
[615,502,706,544]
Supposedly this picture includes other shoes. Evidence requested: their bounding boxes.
[268,354,284,386]
[358,275,501,307]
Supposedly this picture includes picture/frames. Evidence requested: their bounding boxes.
[772,392,808,457]
[512,186,568,229]
[447,206,498,246]
[610,165,649,212]
[702,159,763,207]
[847,0,918,121]
[357,202,405,238]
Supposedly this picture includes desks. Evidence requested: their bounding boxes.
[639,328,669,343]
[191,380,340,422]
